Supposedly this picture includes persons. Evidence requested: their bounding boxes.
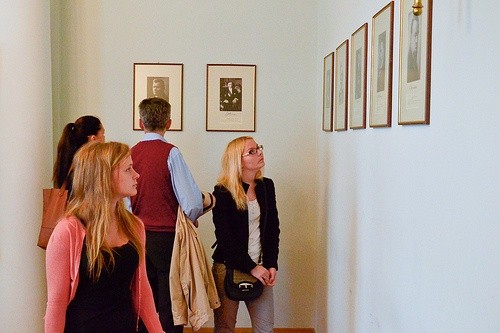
[152,79,169,101]
[211,136,281,333]
[52,115,105,201]
[125,97,216,333]
[220,80,242,111]
[44,141,166,333]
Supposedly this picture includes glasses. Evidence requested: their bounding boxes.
[241,145,263,157]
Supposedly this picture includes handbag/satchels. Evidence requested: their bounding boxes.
[37,163,74,250]
[223,264,263,301]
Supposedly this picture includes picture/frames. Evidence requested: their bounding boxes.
[348,24,369,129]
[133,62,184,131]
[321,52,334,131]
[368,0,395,128]
[206,64,258,133]
[398,0,433,125]
[332,39,350,131]
